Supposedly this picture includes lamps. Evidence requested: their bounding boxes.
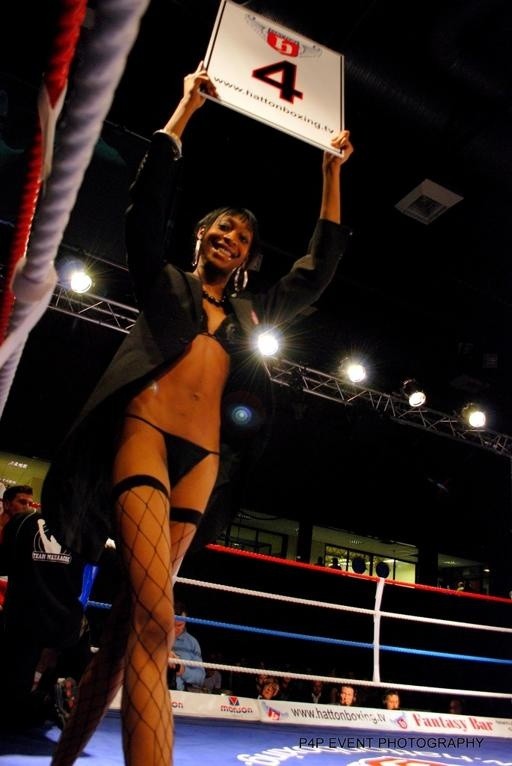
[402,379,426,408]
[461,402,487,429]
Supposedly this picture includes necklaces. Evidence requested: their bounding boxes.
[197,286,230,306]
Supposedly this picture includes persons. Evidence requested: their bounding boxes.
[1,484,512,740]
[51,58,353,766]
